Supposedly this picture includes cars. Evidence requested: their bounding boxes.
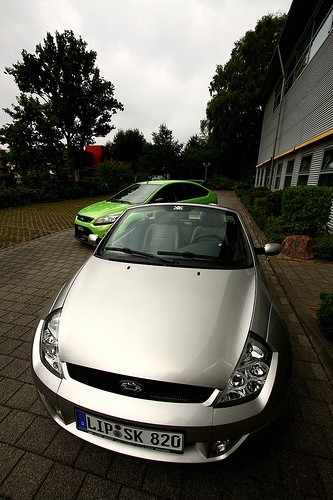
[30,204,293,464]
[74,180,218,246]
